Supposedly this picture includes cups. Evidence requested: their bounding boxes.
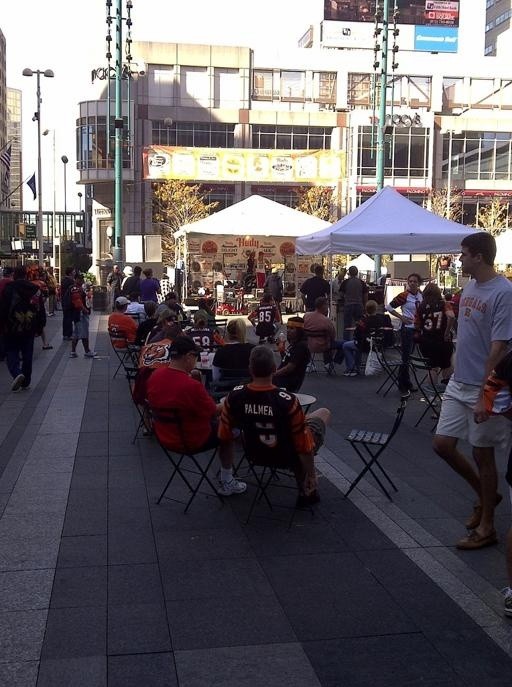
[200,352,208,356]
[209,353,215,365]
[201,356,209,368]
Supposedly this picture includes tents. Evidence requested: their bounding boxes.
[453,230,512,268]
[173,195,333,305]
[343,253,386,278]
[294,186,494,322]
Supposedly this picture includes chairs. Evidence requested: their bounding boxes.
[373,343,410,396]
[134,402,234,514]
[345,400,406,503]
[228,413,316,531]
[108,328,142,379]
[407,355,447,431]
[303,328,339,376]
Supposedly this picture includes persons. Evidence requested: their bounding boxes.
[144,301,157,319]
[39,271,49,314]
[272,316,309,391]
[61,267,76,341]
[440,287,464,310]
[107,264,124,313]
[154,292,178,319]
[120,267,134,290]
[145,338,247,496]
[219,346,329,511]
[123,266,142,302]
[414,283,455,393]
[0,267,47,392]
[38,266,46,282]
[69,273,98,359]
[434,233,512,550]
[385,274,423,392]
[47,266,57,316]
[138,267,161,304]
[300,266,331,316]
[331,268,347,295]
[139,324,182,436]
[182,309,224,353]
[145,310,177,346]
[253,252,271,289]
[108,296,137,349]
[342,301,385,376]
[197,298,215,318]
[27,270,53,349]
[248,293,281,344]
[303,296,347,376]
[339,266,368,341]
[212,319,256,392]
[474,351,512,617]
[248,252,257,273]
[0,267,14,292]
[263,267,283,325]
[124,292,148,324]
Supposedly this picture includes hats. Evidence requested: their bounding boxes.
[115,296,132,307]
[272,268,278,275]
[169,335,204,357]
[155,308,175,323]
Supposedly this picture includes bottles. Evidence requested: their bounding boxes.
[278,333,285,352]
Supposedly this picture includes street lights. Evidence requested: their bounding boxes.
[22,67,54,267]
[62,155,69,241]
[77,192,83,243]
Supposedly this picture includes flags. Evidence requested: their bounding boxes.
[0,139,11,184]
[27,173,36,200]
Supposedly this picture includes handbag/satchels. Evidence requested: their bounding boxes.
[364,336,388,377]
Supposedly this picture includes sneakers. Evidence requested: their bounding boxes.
[83,350,97,359]
[11,374,25,391]
[503,586,512,617]
[305,364,316,374]
[401,386,418,392]
[324,362,334,376]
[23,384,31,390]
[218,478,247,496]
[69,351,79,359]
[297,493,320,509]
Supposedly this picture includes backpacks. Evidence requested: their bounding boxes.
[62,285,82,321]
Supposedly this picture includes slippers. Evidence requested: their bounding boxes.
[43,343,54,350]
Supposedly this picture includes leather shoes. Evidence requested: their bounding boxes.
[464,492,503,529]
[455,529,498,551]
